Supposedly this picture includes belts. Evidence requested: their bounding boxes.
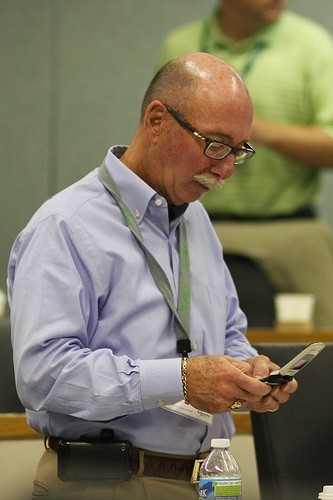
[48,438,202,485]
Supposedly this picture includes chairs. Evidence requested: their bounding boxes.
[252,345,333,500]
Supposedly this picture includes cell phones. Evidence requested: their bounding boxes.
[259,342,326,386]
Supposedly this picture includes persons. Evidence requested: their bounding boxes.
[6,53,297,500]
[150,0,333,219]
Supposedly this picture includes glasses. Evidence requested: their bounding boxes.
[165,103,255,165]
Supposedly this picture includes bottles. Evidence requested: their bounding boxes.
[199,438,244,500]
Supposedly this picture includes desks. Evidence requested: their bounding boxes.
[1,410,260,500]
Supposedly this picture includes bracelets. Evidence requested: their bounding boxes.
[182,357,189,405]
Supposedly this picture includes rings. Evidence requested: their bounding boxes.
[230,399,242,410]
[264,405,280,412]
